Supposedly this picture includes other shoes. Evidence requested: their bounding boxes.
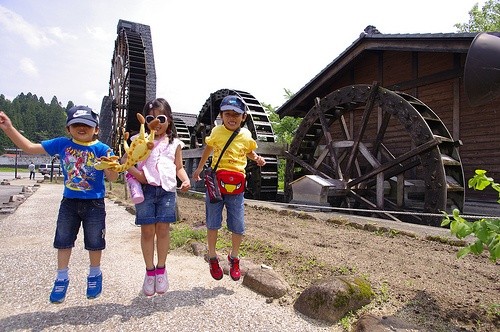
[155,270,169,294]
[144,275,156,296]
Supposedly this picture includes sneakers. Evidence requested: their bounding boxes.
[86,270,102,299]
[228,251,240,280]
[208,253,223,280]
[50,278,69,304]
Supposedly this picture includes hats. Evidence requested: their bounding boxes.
[66,106,98,127]
[221,95,246,114]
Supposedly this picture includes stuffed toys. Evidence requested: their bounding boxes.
[95,111,159,171]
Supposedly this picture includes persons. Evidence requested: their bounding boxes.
[193,96,266,281]
[0,106,119,304]
[29,162,35,180]
[122,98,191,297]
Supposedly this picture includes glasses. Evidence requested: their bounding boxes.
[145,115,169,125]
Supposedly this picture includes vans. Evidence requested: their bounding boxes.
[38,163,62,176]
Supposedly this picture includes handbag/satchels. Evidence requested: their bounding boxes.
[215,170,247,195]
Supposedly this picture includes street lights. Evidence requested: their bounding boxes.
[15,129,25,178]
